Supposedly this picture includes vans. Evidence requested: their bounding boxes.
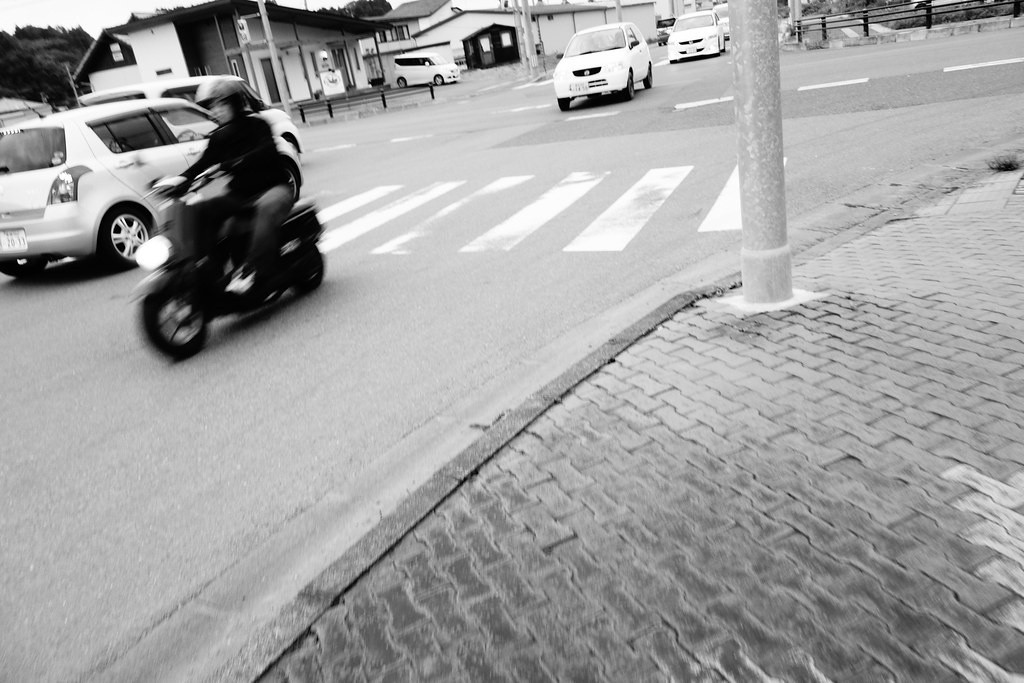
[394,51,461,89]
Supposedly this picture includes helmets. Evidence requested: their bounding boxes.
[196,76,241,104]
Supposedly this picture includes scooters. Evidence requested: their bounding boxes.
[132,158,327,364]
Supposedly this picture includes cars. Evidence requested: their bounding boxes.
[666,11,726,66]
[0,96,308,285]
[710,1,730,43]
[552,20,655,112]
[71,73,304,157]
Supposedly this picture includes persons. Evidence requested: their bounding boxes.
[150,74,294,295]
[613,32,626,49]
